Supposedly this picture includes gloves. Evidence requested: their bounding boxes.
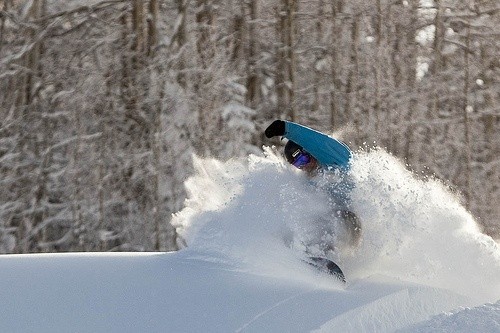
[265,120,285,139]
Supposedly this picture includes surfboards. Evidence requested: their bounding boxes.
[304,257,346,283]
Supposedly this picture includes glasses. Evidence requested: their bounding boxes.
[291,155,311,169]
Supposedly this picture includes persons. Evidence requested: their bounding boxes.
[265,120,364,261]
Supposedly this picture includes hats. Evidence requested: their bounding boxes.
[283,140,310,165]
